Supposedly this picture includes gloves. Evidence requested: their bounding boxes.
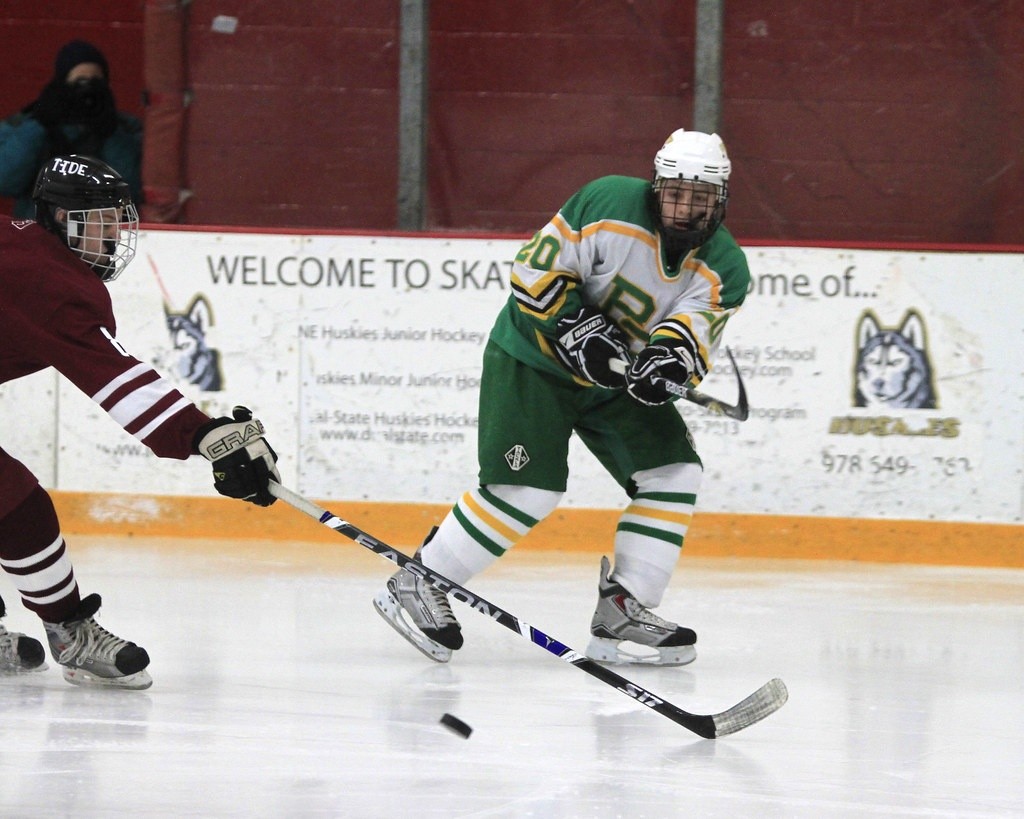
[81,78,118,144]
[192,405,281,507]
[557,306,632,389]
[28,75,86,127]
[627,337,696,406]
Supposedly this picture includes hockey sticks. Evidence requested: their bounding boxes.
[267,480,790,743]
[607,347,750,424]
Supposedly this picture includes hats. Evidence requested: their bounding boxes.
[54,38,109,83]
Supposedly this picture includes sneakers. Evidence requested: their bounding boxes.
[588,555,697,667]
[373,525,463,662]
[42,592,153,691]
[0,625,50,676]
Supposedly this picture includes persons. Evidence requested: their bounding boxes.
[374,129,750,664]
[0,154,281,690]
[0,40,144,223]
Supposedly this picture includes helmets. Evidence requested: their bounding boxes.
[31,154,140,283]
[650,128,733,250]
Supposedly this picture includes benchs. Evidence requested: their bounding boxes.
[0,1,1024,249]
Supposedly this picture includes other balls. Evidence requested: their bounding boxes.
[440,713,472,739]
